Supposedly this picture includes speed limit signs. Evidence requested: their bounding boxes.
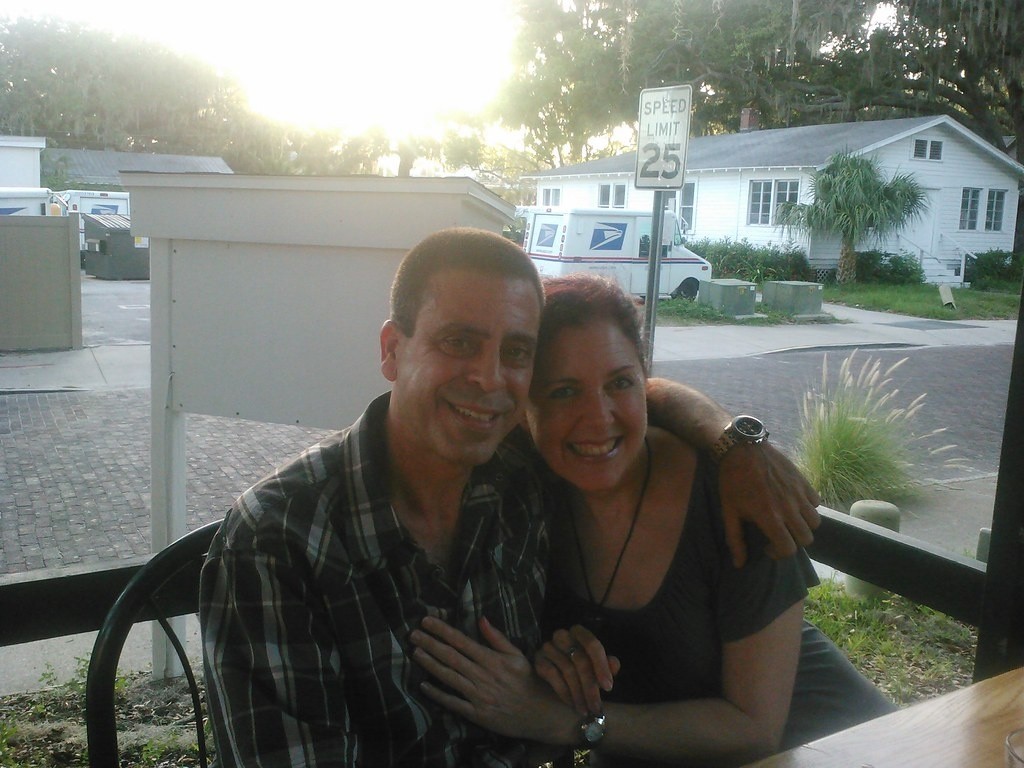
[635,82,692,190]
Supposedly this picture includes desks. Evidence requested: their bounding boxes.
[742,665,1024,768]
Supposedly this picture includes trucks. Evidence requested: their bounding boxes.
[522,205,711,302]
[0,188,130,269]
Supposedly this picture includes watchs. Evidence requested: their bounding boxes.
[577,704,606,751]
[709,414,771,467]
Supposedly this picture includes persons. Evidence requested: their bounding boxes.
[195,228,820,768]
[408,273,898,768]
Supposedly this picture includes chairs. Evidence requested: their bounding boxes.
[85,516,235,767]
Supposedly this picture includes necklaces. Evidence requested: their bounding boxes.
[566,438,653,620]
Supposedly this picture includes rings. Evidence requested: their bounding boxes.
[567,643,583,662]
